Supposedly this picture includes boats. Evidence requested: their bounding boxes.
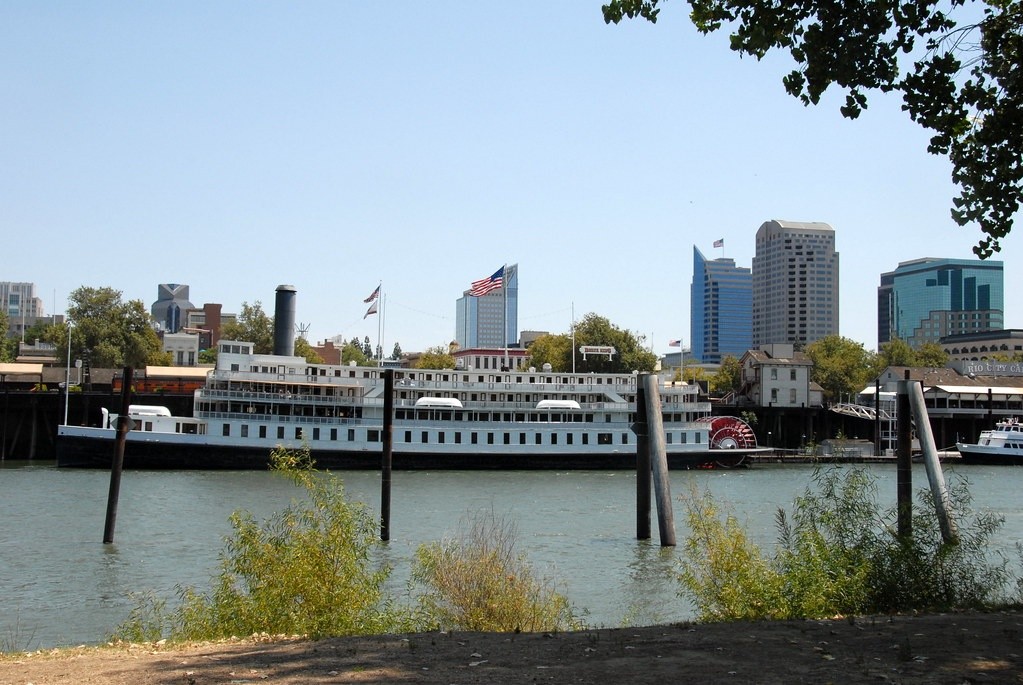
[58,338,773,471]
[955,417,1023,465]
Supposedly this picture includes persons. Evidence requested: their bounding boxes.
[279,389,291,398]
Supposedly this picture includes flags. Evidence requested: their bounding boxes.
[713,239,723,248]
[364,300,377,319]
[469,265,504,297]
[669,340,680,347]
[363,286,380,303]
